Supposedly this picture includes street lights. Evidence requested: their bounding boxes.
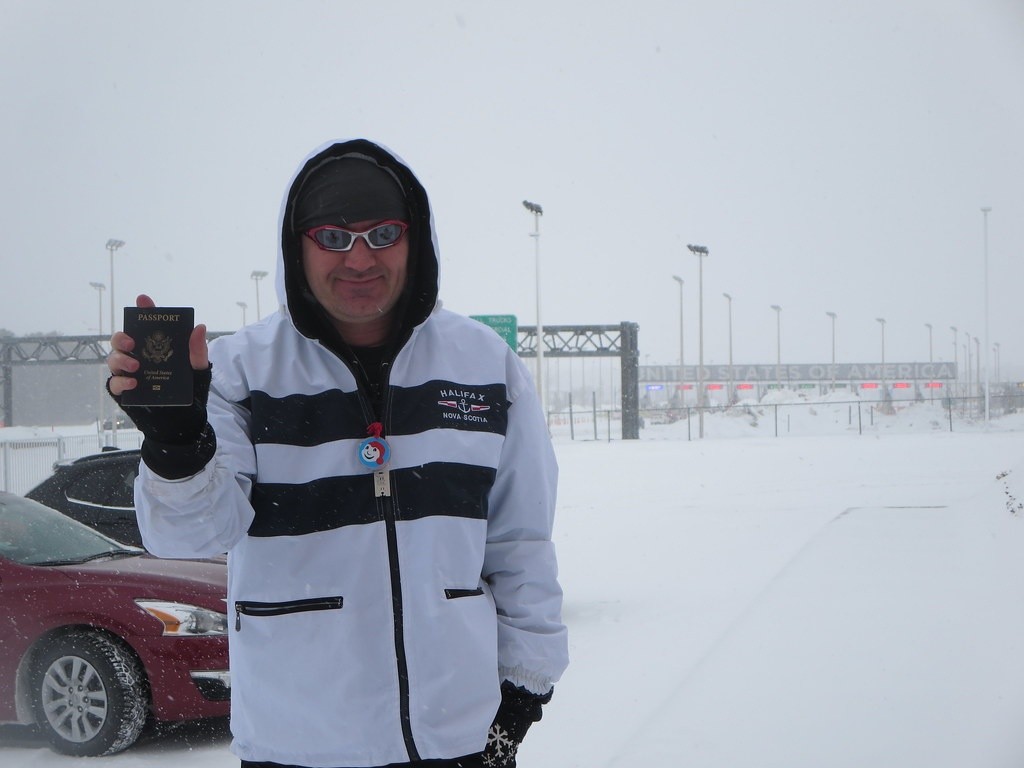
[724,293,732,410]
[673,274,689,428]
[239,301,249,324]
[980,206,993,421]
[875,318,885,364]
[951,327,1000,407]
[250,270,268,322]
[688,244,709,439]
[524,199,545,420]
[924,324,932,363]
[828,311,837,367]
[89,282,105,335]
[106,238,125,334]
[771,304,781,384]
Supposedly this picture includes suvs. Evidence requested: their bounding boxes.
[21,444,229,556]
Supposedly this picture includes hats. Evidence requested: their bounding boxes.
[294,156,411,234]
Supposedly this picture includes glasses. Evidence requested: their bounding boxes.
[297,219,408,253]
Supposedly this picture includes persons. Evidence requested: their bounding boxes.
[106,139,570,768]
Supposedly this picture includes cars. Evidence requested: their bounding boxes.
[0,489,236,757]
